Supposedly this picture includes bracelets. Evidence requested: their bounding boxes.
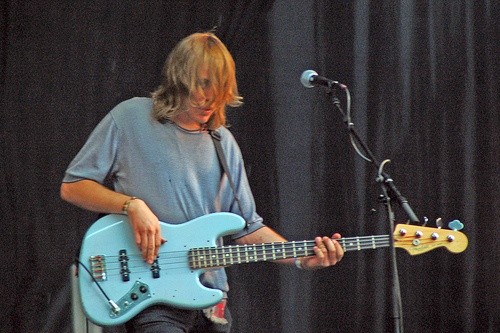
[121,196,137,216]
[295,257,303,270]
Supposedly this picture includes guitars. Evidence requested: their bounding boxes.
[75,206,469,326]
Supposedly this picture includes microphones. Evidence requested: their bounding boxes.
[300,69,348,91]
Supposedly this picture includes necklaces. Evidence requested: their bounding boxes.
[173,120,205,132]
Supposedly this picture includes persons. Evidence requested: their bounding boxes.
[60,32,344,333]
